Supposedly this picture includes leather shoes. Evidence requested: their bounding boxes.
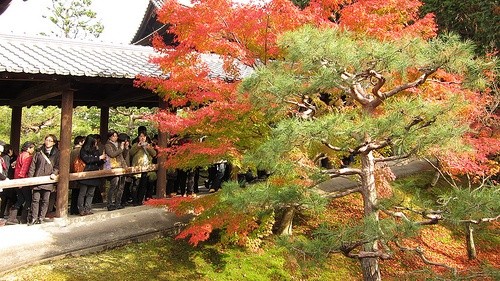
[28,218,44,226]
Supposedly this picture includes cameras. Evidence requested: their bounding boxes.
[141,142,147,145]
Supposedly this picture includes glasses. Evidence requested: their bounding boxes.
[45,139,54,143]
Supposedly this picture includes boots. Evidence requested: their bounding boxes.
[5,209,19,225]
[22,211,31,224]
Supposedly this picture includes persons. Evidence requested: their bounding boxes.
[0,127,253,227]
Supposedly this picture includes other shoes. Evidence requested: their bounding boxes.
[108,203,123,210]
[182,192,196,198]
[80,210,93,215]
[133,202,143,206]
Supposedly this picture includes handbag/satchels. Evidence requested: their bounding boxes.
[70,149,86,173]
[148,161,157,183]
[102,144,119,168]
